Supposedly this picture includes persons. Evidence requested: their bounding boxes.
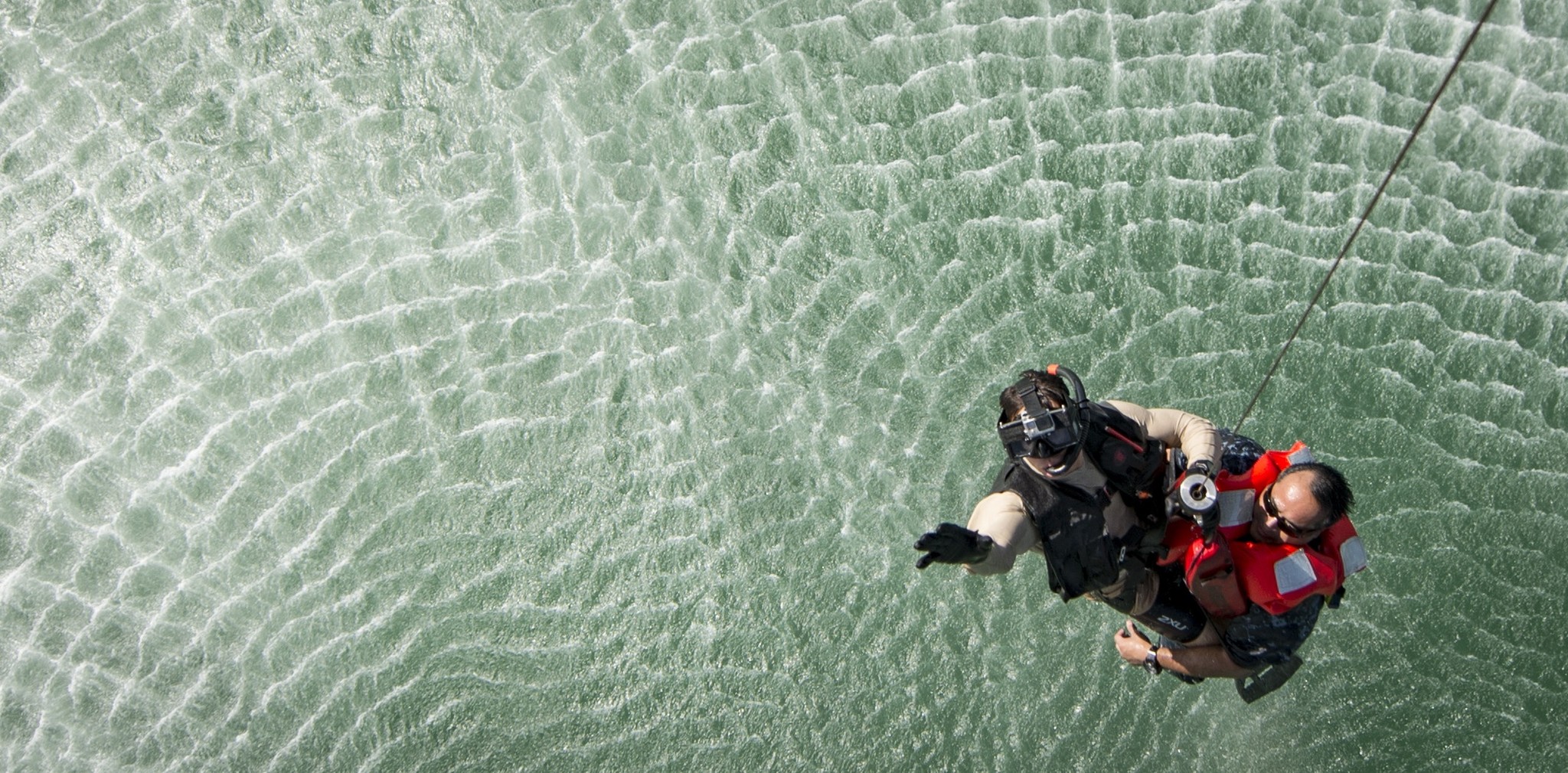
[1115,439,1364,686]
[913,364,1226,648]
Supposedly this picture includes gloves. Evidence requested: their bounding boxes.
[913,524,991,568]
[1165,460,1223,542]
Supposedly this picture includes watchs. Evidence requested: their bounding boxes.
[1142,645,1162,675]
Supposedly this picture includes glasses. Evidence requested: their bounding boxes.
[1263,482,1322,539]
